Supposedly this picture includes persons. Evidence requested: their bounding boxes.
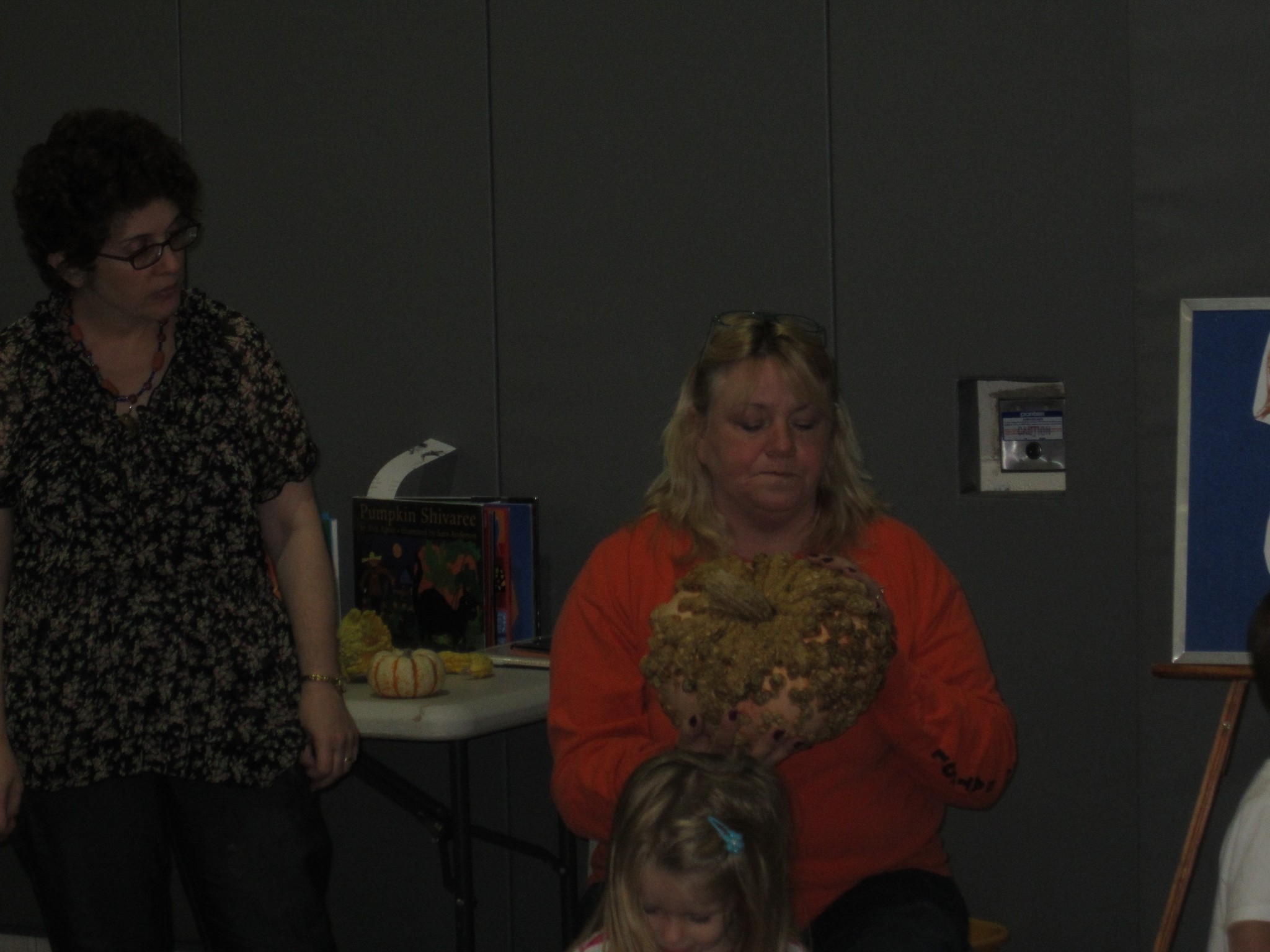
[1205,592,1270,952]
[0,105,361,952]
[548,311,1018,952]
[565,749,805,952]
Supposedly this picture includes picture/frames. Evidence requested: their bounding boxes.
[1171,295,1270,666]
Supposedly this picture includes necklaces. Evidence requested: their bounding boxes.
[63,292,168,429]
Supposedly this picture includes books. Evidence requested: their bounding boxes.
[353,496,547,655]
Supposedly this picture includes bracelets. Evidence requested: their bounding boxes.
[300,673,344,687]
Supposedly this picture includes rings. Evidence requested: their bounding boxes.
[344,757,354,763]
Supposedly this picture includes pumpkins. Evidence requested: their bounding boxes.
[337,608,393,680]
[368,647,446,699]
[642,552,895,750]
[438,650,493,678]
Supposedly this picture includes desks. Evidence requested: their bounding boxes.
[333,661,585,952]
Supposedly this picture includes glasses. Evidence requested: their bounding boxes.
[68,212,202,271]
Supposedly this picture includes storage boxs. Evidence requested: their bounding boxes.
[353,496,543,654]
